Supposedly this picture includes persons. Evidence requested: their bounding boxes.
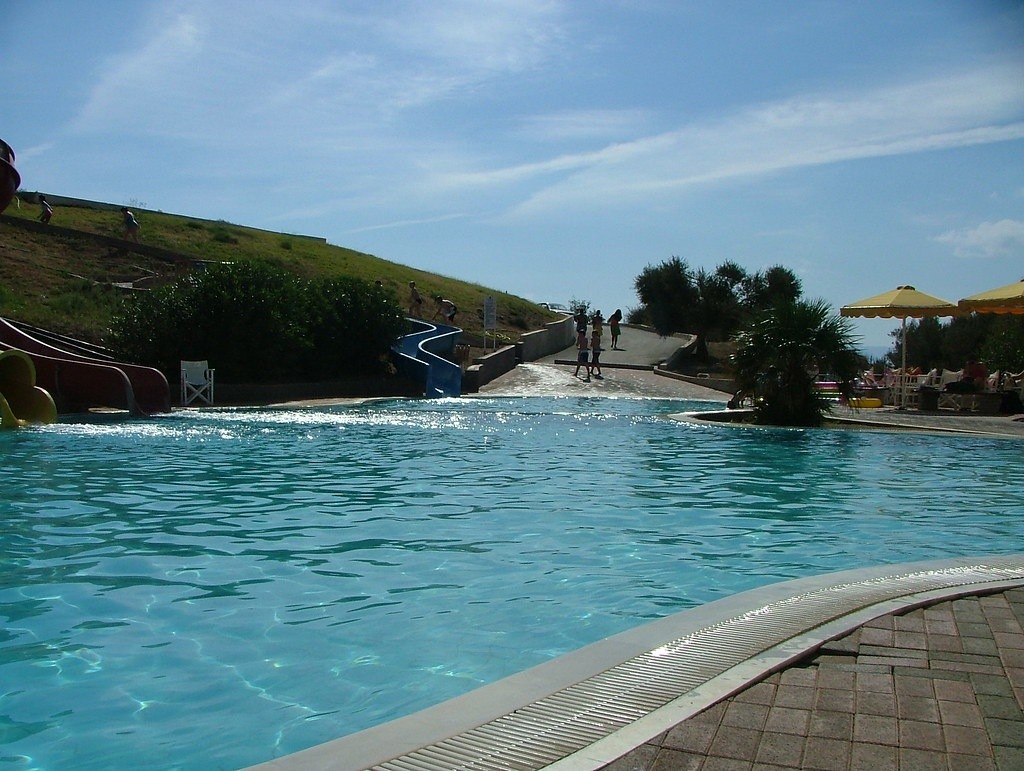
[590,331,602,375]
[574,308,589,348]
[37,195,53,223]
[432,296,457,325]
[1011,370,1024,407]
[120,207,141,243]
[592,310,603,346]
[407,281,422,319]
[608,309,622,349]
[573,329,590,382]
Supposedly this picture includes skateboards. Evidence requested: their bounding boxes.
[0,312,175,427]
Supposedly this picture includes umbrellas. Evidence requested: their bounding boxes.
[840,286,958,407]
[958,279,1024,314]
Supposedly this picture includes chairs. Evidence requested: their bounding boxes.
[882,365,1023,411]
[180,360,215,407]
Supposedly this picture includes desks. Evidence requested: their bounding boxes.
[850,387,890,398]
[913,388,1009,416]
